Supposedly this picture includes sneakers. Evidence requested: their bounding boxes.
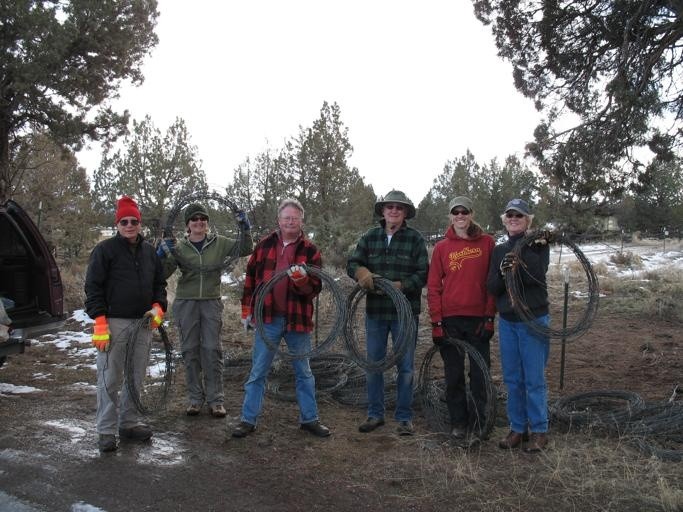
[499,429,528,453]
[450,420,469,440]
[358,415,386,433]
[396,418,414,437]
[208,399,227,418]
[98,431,119,453]
[525,432,548,453]
[186,402,205,418]
[118,423,154,444]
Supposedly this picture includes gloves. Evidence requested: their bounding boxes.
[91,315,112,353]
[156,236,178,258]
[144,303,166,332]
[354,265,378,290]
[240,305,256,336]
[287,261,310,288]
[474,319,495,341]
[499,253,520,278]
[376,281,400,296]
[234,208,250,232]
[431,324,444,343]
[529,229,551,247]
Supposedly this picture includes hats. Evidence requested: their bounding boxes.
[114,194,142,224]
[448,195,474,212]
[375,191,416,219]
[506,199,532,218]
[184,203,209,224]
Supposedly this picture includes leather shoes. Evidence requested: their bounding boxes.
[300,419,330,437]
[232,421,257,438]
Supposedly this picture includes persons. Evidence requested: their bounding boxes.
[344,190,429,435]
[425,195,495,441]
[82,195,169,453]
[229,198,331,438]
[486,198,549,454]
[154,202,255,418]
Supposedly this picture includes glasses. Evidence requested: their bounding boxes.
[451,210,470,215]
